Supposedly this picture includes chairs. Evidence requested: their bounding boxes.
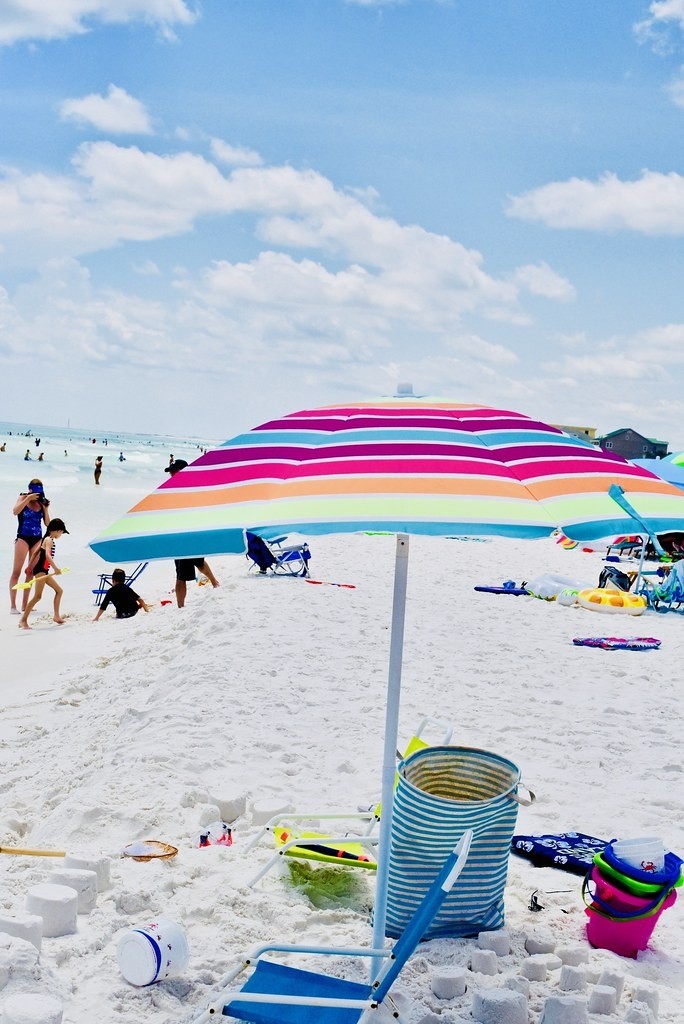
[89,557,152,606]
[605,533,644,557]
[240,716,454,889]
[190,826,475,1024]
[238,529,310,582]
[633,535,656,561]
[637,572,684,613]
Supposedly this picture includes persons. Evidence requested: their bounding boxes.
[1,427,684,629]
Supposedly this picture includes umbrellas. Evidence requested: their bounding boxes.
[82,383,684,984]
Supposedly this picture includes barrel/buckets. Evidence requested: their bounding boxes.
[581,836,684,961]
[116,917,190,987]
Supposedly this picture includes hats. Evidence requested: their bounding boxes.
[165,460,188,472]
[29,484,43,493]
[48,519,69,534]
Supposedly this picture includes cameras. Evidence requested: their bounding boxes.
[36,492,49,507]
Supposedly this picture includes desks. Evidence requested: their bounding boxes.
[626,570,667,588]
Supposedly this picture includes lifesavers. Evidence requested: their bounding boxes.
[577,588,648,617]
[557,527,649,552]
[524,573,595,607]
